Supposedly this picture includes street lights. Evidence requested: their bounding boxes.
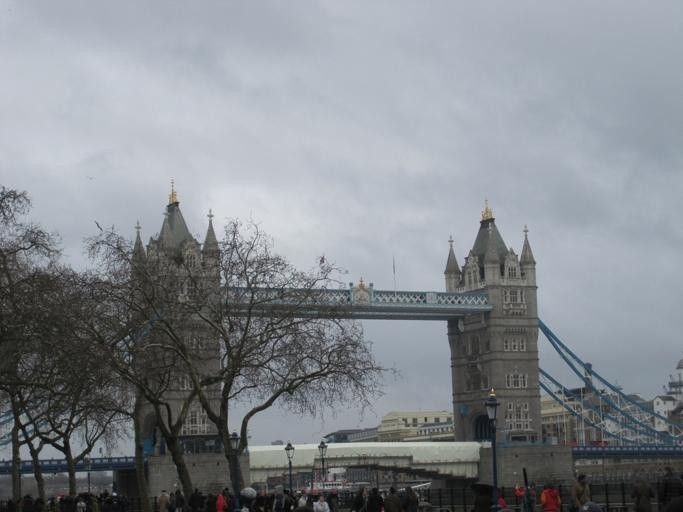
[483,386,500,499]
[228,430,241,509]
[83,458,93,496]
[284,441,294,495]
[317,440,328,481]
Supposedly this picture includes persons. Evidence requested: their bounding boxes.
[1,482,129,512]
[158,485,338,512]
[355,486,418,512]
[630,467,673,512]
[496,475,590,512]
